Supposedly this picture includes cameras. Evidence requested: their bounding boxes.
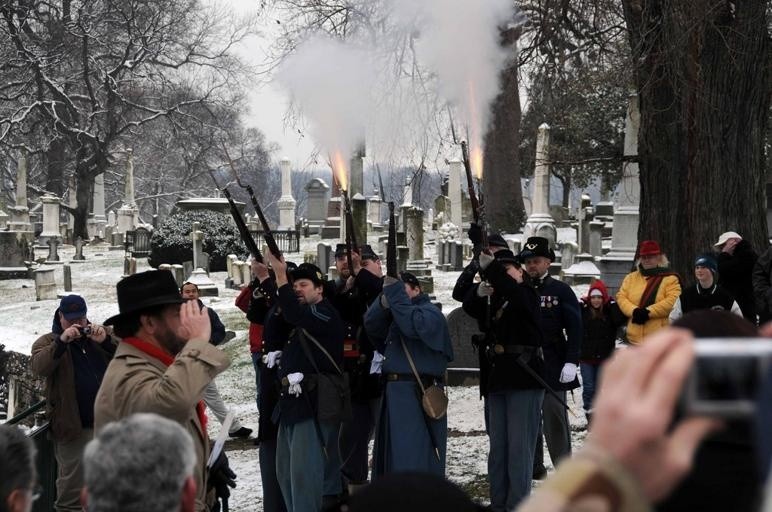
[77,326,91,334]
[685,338,772,414]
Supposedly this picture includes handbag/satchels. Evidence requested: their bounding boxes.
[291,317,353,422]
[396,333,449,420]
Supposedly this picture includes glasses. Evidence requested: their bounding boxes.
[66,317,88,325]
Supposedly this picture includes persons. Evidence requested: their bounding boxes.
[615,241,681,346]
[339,243,385,478]
[251,258,297,511]
[668,295,683,325]
[177,282,253,438]
[714,231,743,268]
[578,280,630,425]
[327,243,349,308]
[93,269,237,512]
[462,250,546,512]
[513,322,772,512]
[0,423,36,512]
[31,295,121,511]
[364,272,455,477]
[234,260,266,373]
[750,238,772,327]
[346,470,489,512]
[79,412,197,512]
[669,255,744,324]
[451,224,511,331]
[648,311,772,512]
[269,250,339,512]
[516,236,580,480]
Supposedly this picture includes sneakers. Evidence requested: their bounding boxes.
[228,427,253,437]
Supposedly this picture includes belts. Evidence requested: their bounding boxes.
[488,343,542,358]
[380,373,445,385]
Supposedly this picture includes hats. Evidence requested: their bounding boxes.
[714,232,742,247]
[334,243,348,257]
[513,236,556,263]
[289,262,323,287]
[695,257,719,284]
[100,269,195,326]
[356,244,379,259]
[491,250,521,267]
[487,233,510,248]
[60,295,87,319]
[637,240,660,255]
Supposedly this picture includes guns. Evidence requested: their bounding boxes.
[244,186,281,262]
[221,186,263,264]
[343,190,360,278]
[460,141,497,285]
[387,202,397,279]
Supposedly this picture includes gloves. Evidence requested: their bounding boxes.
[632,307,651,326]
[558,363,578,383]
[380,294,393,308]
[382,275,403,287]
